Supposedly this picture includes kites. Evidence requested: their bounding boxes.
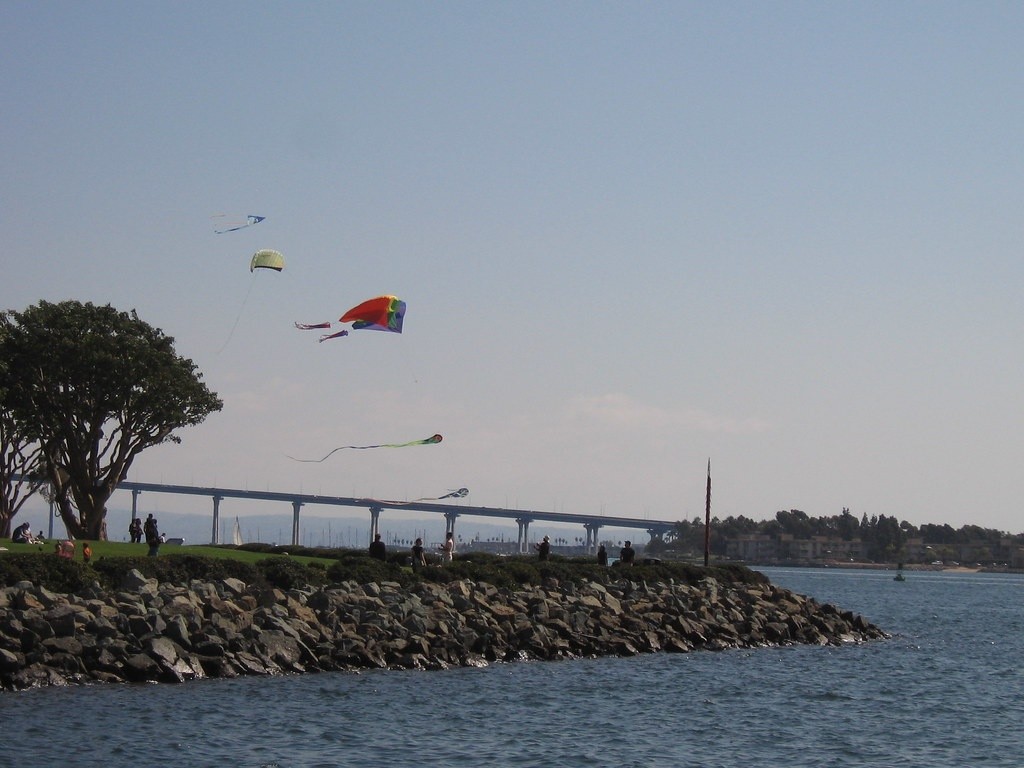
[286,434,443,462]
[212,216,267,234]
[368,488,469,505]
[295,295,406,342]
[249,249,285,274]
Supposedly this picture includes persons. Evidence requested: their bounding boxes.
[620,541,635,567]
[411,538,426,573]
[439,532,453,566]
[129,518,143,543]
[370,534,385,563]
[55,540,63,554]
[598,546,608,567]
[12,522,46,545]
[83,543,93,563]
[534,535,550,563]
[144,514,160,557]
[158,532,166,544]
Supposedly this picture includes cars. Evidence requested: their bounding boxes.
[932,561,943,564]
[948,561,959,566]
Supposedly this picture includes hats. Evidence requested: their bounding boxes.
[625,541,631,544]
